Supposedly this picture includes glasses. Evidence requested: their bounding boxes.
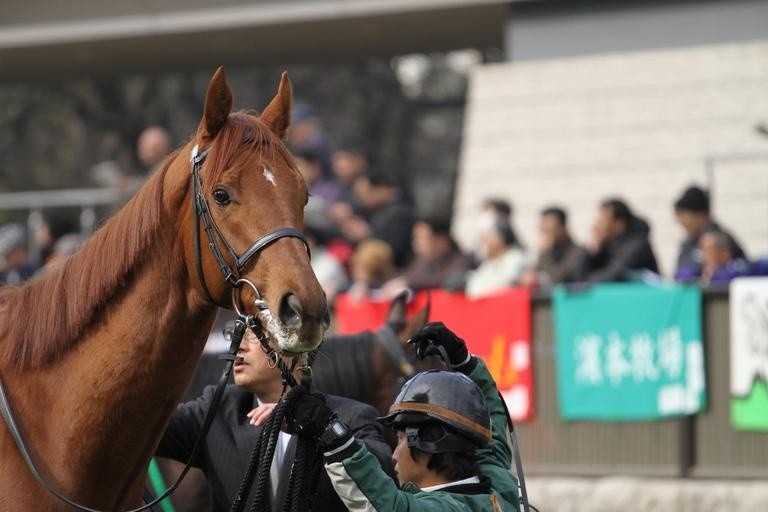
[222,327,235,340]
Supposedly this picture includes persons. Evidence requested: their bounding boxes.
[153,308,393,512]
[245,321,527,512]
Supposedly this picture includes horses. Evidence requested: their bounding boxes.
[0,64,332,512]
[141,289,432,512]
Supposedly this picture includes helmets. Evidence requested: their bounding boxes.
[372,367,492,449]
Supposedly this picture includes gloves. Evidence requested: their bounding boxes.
[281,392,336,442]
[405,321,472,372]
